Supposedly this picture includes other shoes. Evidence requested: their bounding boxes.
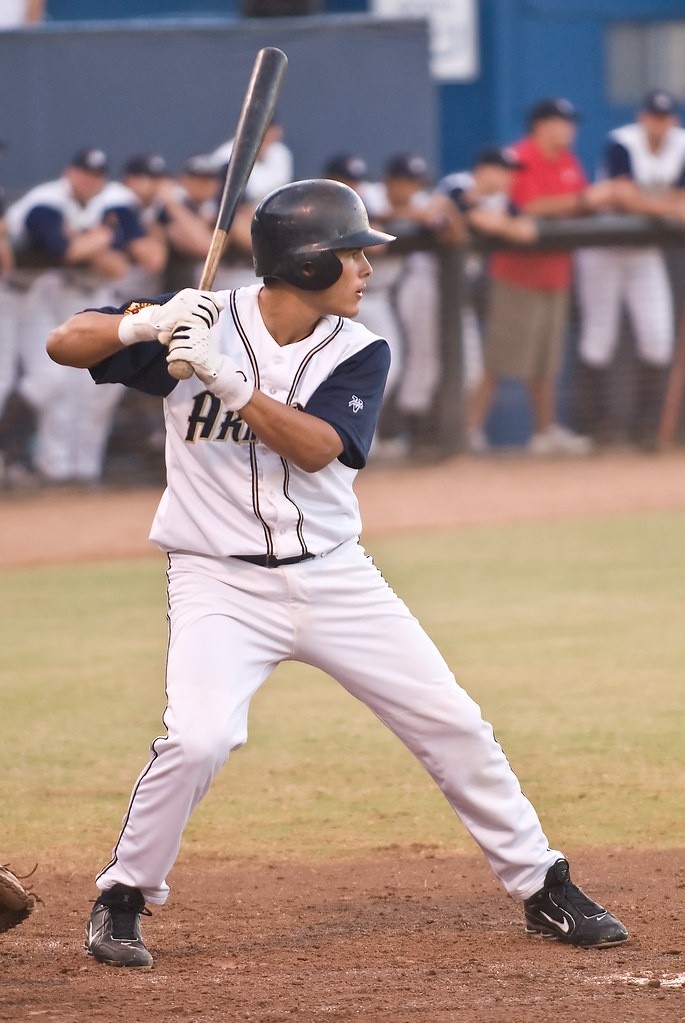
[527,424,593,457]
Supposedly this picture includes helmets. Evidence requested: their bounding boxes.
[250,178,397,291]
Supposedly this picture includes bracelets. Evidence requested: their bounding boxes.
[575,188,591,211]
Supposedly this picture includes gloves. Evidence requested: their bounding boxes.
[119,287,224,348]
[165,322,255,413]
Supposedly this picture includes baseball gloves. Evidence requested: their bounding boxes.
[0,859,47,933]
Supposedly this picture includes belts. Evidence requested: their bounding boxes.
[229,553,314,568]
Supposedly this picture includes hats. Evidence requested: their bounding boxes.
[129,154,168,174]
[530,98,577,118]
[386,153,430,178]
[185,154,228,181]
[70,148,106,172]
[643,92,677,113]
[477,146,524,168]
[326,152,366,182]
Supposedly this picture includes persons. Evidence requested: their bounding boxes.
[0,111,543,490]
[462,95,618,456]
[46,178,631,963]
[564,88,685,451]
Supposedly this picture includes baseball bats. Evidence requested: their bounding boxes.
[167,42,289,383]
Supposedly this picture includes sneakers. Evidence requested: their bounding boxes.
[83,884,156,969]
[524,858,629,950]
